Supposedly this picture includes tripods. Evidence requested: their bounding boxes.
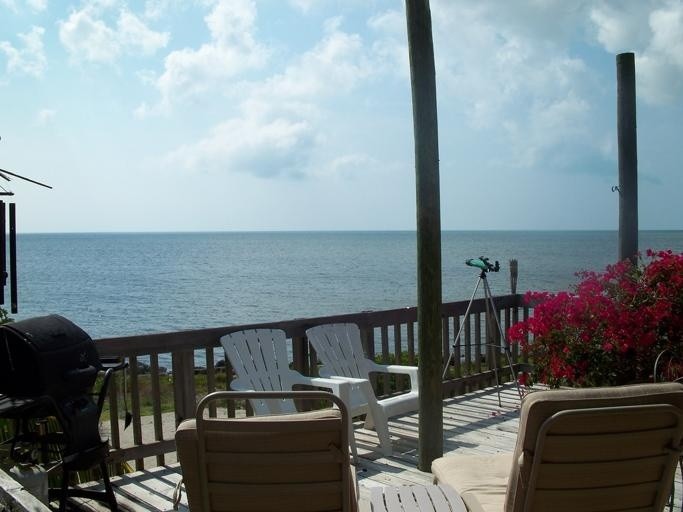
[444,276,522,407]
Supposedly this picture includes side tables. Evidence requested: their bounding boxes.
[359,483,467,511]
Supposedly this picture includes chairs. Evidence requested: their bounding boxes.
[0,364,119,512]
[431,382,683,512]
[173,387,360,512]
[218,328,359,468]
[305,324,421,460]
